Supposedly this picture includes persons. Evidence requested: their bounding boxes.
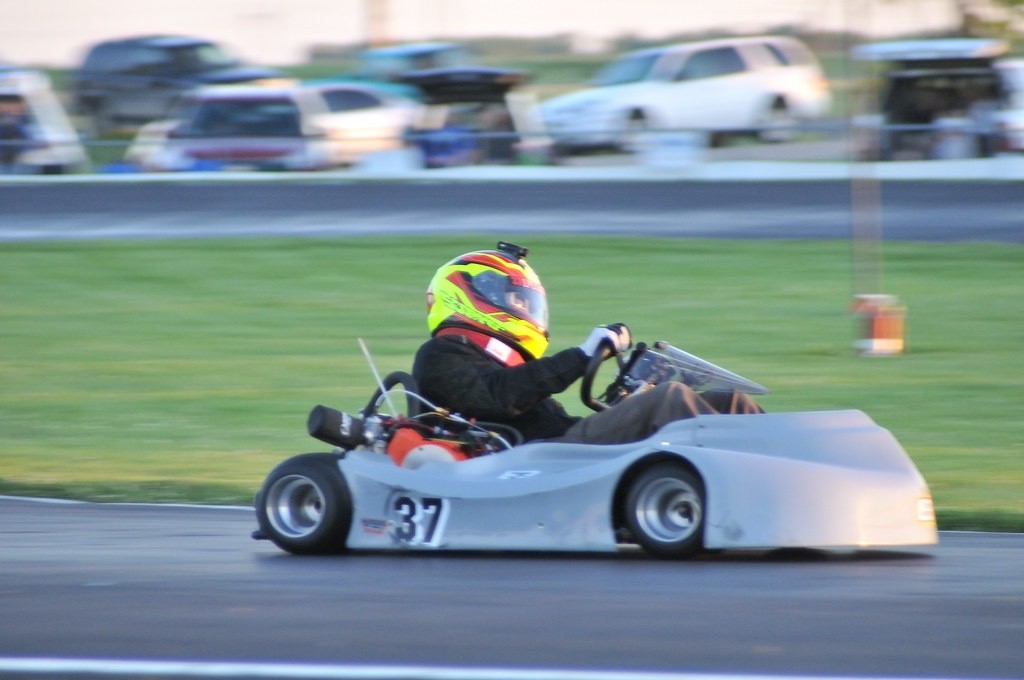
[412,241,766,446]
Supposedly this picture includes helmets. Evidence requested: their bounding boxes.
[426,241,549,361]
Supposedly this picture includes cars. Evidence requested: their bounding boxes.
[354,42,530,99]
[531,28,829,160]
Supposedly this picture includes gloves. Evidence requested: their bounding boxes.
[576,323,633,358]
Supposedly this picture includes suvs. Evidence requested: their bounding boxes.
[63,28,276,127]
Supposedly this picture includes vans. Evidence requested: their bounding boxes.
[130,82,434,172]
